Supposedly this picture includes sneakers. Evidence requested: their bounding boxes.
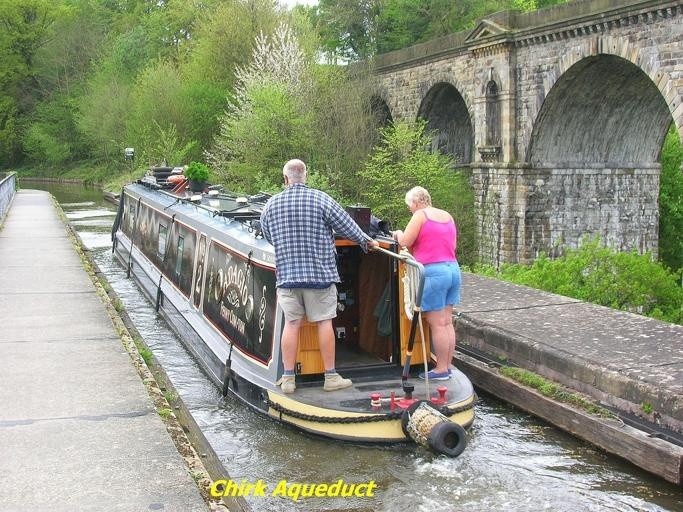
[418,368,452,380]
[277,374,296,393]
[324,373,352,391]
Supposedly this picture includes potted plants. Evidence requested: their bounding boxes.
[186,161,207,193]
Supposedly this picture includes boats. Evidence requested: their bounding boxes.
[110,161,480,458]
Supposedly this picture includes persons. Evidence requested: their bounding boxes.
[258,158,381,396]
[385,183,464,382]
